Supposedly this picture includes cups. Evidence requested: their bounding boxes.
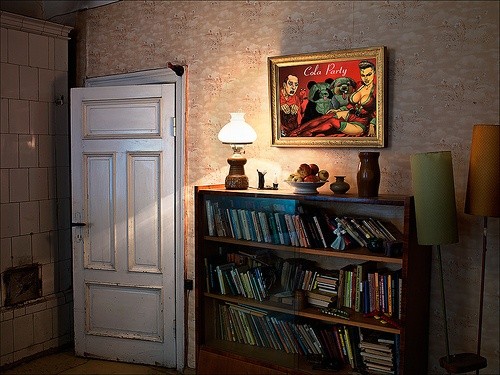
[358,151,381,197]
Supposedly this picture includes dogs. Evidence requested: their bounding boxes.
[331,77,357,110]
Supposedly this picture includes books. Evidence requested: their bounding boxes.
[205,199,403,375]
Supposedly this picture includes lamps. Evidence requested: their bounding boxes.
[464,125,500,375]
[218,112,257,189]
[410,151,460,375]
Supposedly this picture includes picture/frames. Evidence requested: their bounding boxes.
[268,45,388,149]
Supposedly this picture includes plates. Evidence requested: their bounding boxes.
[284,180,326,193]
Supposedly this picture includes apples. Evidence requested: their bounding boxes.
[287,163,329,182]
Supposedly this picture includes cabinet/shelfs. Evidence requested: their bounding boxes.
[193,183,431,375]
